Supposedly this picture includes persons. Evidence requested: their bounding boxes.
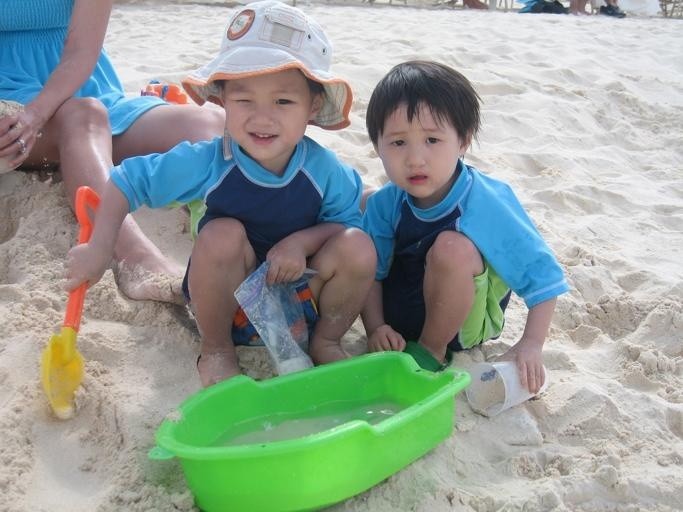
[61,0,378,389]
[355,61,568,396]
[0,0,224,305]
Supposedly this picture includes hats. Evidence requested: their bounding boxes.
[180,0,353,132]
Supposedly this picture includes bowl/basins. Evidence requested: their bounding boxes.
[144,351,473,512]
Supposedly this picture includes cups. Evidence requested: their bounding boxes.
[464,361,547,418]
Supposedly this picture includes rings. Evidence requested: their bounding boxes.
[17,137,26,150]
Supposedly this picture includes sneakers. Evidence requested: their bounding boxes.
[599,5,627,18]
